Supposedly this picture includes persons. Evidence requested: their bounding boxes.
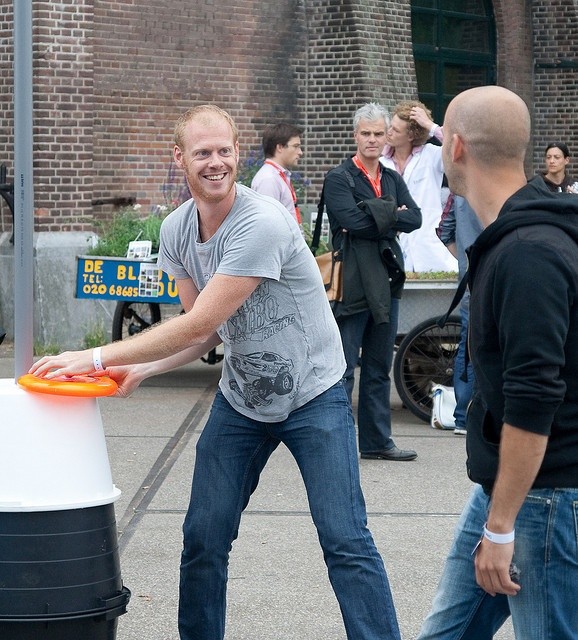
[377,99,458,275]
[535,140,578,194]
[411,82,577,639]
[249,121,304,227]
[30,101,403,640]
[316,103,424,462]
[433,190,485,436]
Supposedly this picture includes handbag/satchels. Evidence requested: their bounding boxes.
[430,384,458,430]
[313,249,344,302]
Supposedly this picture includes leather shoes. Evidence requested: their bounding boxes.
[360,445,418,461]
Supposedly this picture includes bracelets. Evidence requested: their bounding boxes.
[429,124,439,137]
[483,522,518,545]
[92,345,106,372]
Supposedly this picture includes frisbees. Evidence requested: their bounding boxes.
[17,372,118,397]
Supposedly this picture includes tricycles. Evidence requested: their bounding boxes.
[109,221,468,432]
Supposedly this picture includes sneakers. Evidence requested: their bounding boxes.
[454,428,467,435]
